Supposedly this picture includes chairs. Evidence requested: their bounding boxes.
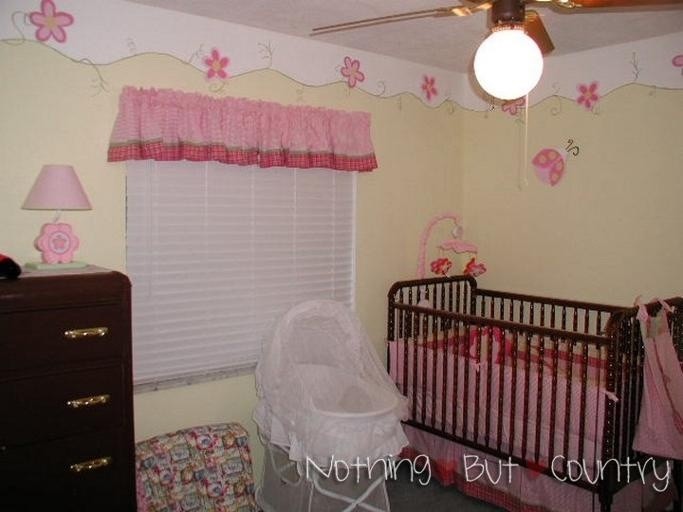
[135,423,264,512]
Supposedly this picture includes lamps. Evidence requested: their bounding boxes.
[474,0,544,100]
[21,164,92,268]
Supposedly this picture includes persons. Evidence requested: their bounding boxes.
[461,325,512,365]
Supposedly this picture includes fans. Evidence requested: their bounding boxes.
[309,0,683,58]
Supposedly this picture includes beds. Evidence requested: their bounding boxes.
[388,275,683,512]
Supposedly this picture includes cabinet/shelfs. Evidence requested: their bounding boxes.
[0,265,138,512]
